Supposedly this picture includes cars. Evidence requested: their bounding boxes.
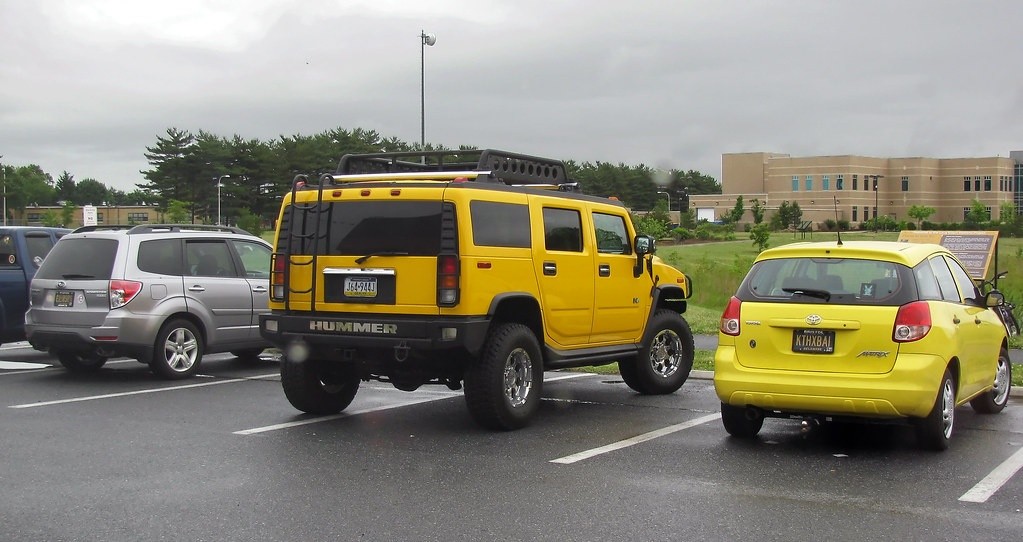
[714,241,1012,453]
[0,225,74,347]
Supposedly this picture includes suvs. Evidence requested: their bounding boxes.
[23,221,275,382]
[255,149,697,434]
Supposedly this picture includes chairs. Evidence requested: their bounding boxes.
[197,256,219,275]
[783,275,843,291]
[871,279,898,299]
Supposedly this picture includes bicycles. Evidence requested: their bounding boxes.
[973,270,1020,341]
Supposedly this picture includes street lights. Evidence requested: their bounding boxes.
[657,192,671,210]
[217,175,232,233]
[419,28,437,164]
[875,184,880,218]
